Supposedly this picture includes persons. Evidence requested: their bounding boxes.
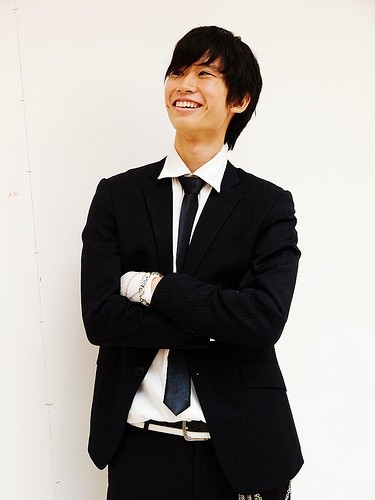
[81,26,304,500]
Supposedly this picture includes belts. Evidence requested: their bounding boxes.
[131,420,211,441]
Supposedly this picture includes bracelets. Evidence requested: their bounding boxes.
[138,272,165,307]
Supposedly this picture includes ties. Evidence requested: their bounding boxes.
[163,175,207,416]
